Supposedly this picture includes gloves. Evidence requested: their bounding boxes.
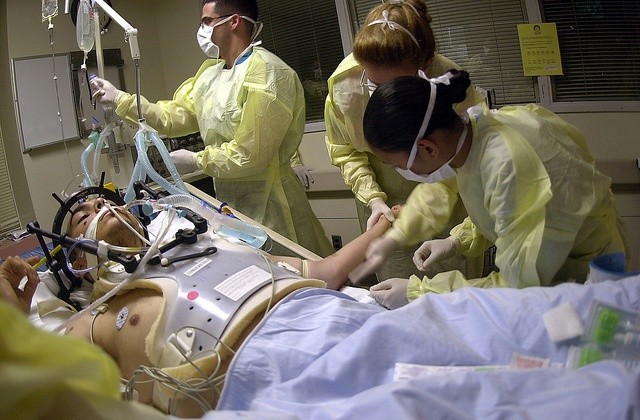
[348,234,399,286]
[89,77,121,104]
[369,277,409,310]
[169,148,198,175]
[412,238,456,272]
[365,198,396,232]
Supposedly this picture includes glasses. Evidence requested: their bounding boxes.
[359,67,378,92]
[199,13,234,28]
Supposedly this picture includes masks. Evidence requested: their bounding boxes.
[394,68,469,184]
[196,13,264,81]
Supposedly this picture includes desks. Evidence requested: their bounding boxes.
[139,167,213,193]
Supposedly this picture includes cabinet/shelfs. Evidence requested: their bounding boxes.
[307,198,362,250]
[610,194,640,272]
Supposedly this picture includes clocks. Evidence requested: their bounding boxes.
[73,0,113,31]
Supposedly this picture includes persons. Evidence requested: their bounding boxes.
[325,1,484,284]
[90,0,336,257]
[1,187,403,418]
[363,69,637,311]
